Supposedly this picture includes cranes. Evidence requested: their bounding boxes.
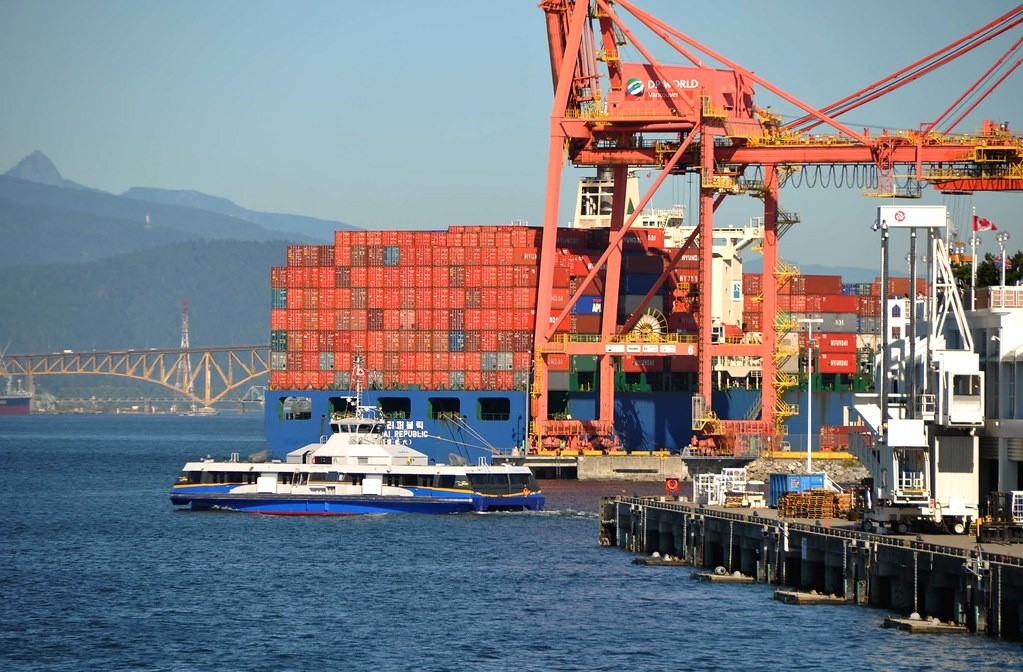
[527,0,1023,453]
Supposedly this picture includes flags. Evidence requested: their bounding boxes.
[972,215,998,231]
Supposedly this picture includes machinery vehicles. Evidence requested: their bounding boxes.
[970,490,1023,547]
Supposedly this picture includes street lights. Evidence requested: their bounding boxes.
[996,230,1010,287]
[904,251,911,278]
[967,236,983,277]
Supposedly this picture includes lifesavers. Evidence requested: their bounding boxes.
[311,457,316,464]
[523,488,530,497]
[667,479,678,490]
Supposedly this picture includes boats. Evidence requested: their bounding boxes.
[170,344,546,516]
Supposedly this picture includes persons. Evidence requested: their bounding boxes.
[901,283,971,299]
